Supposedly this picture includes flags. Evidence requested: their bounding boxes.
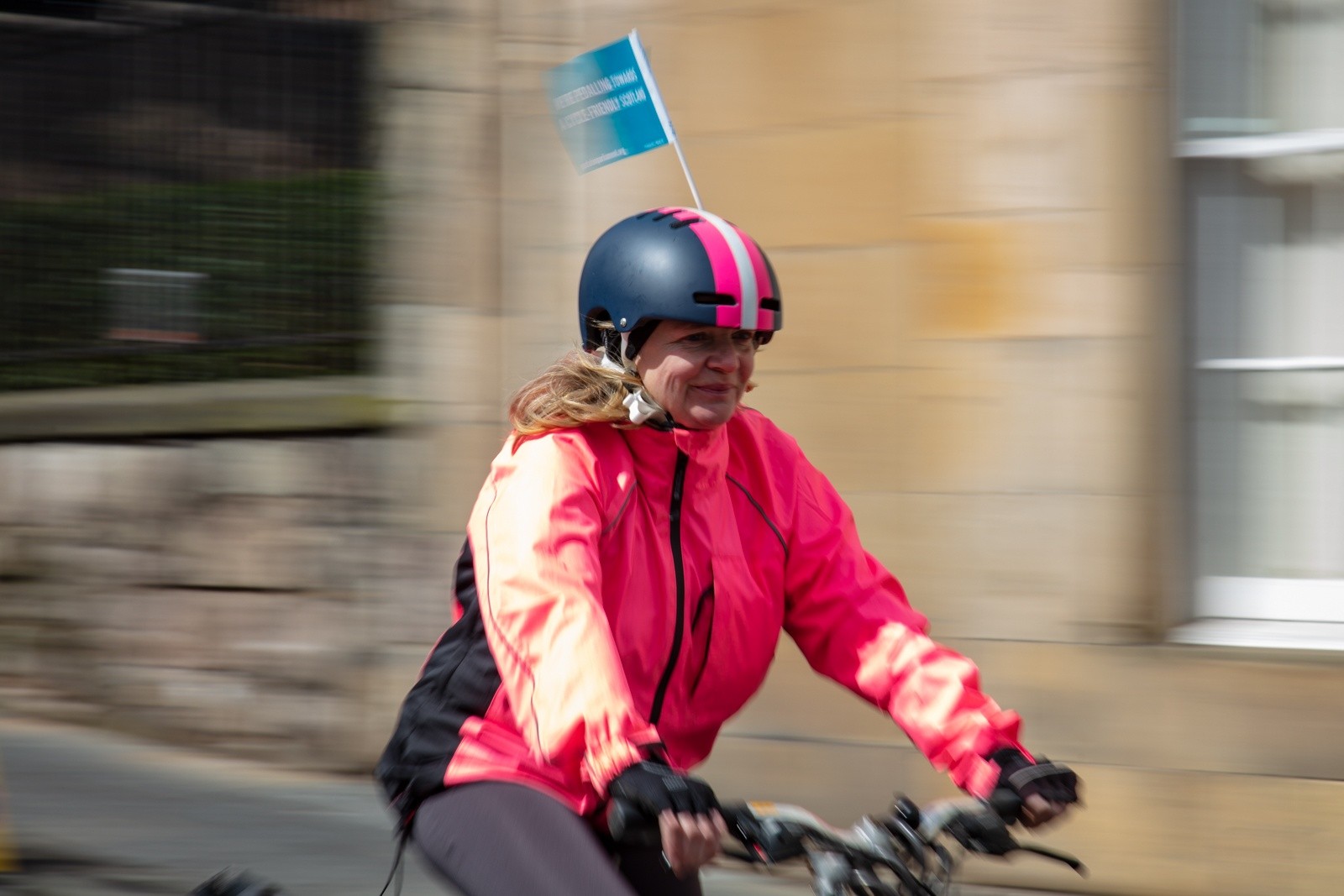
[543,36,673,174]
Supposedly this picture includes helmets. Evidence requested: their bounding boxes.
[578,206,783,360]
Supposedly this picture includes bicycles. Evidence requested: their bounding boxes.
[604,788,1091,896]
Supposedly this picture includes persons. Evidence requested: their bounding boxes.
[377,204,1078,896]
